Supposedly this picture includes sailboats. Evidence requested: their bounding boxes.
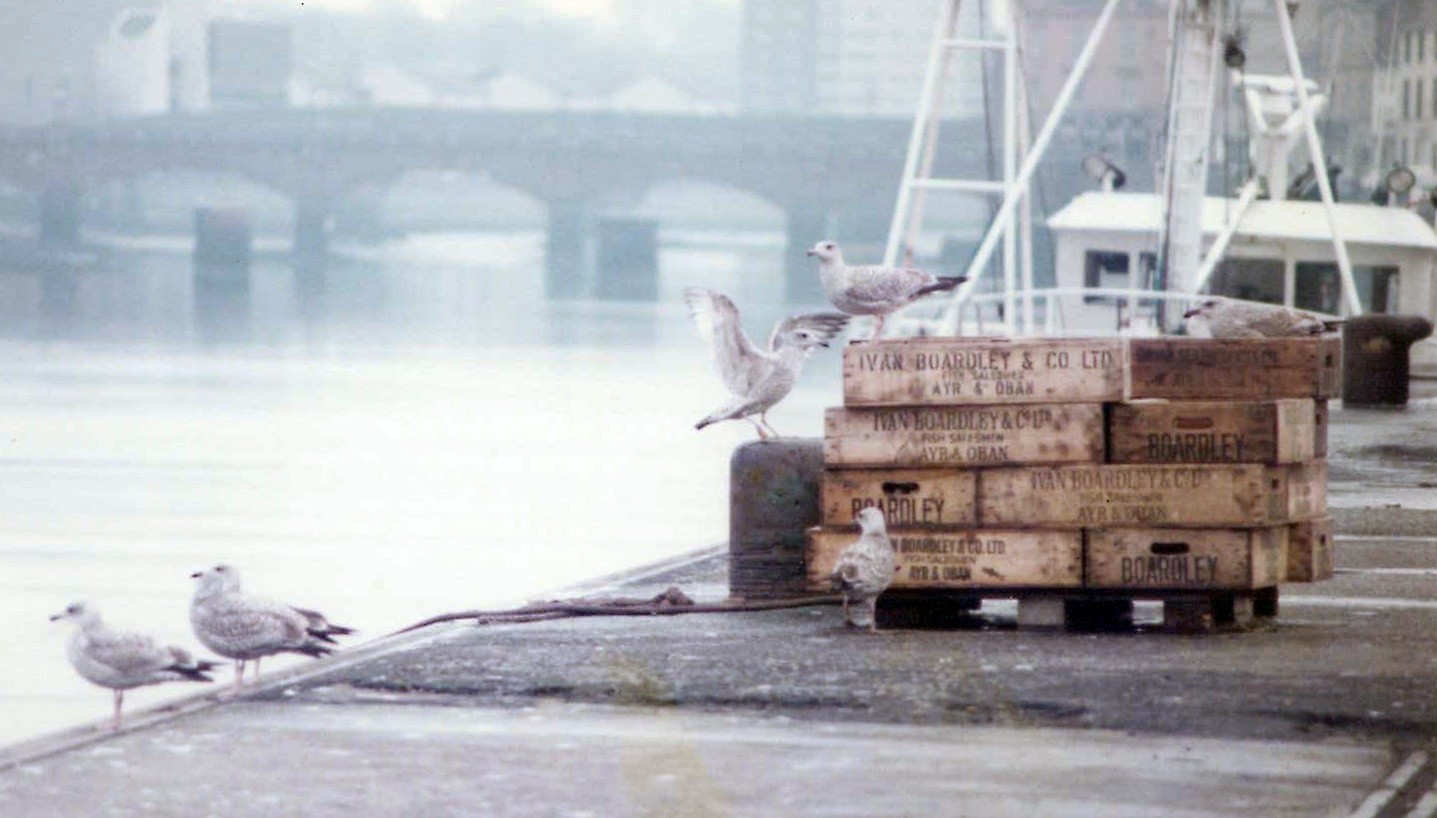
[864,0,1437,407]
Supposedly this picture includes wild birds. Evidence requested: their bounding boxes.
[50,597,227,731]
[1183,296,1348,340]
[829,507,897,636]
[189,563,361,700]
[680,241,972,444]
